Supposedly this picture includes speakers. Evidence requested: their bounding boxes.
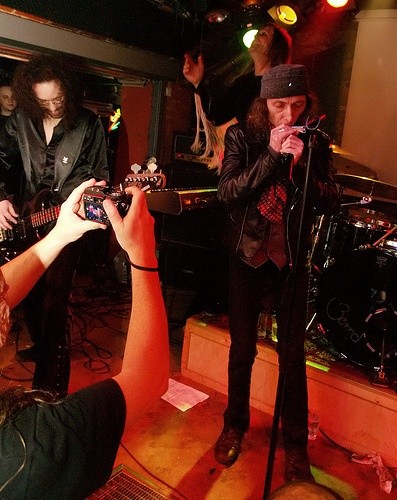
[162,164,222,252]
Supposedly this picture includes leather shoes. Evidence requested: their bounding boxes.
[215,427,244,466]
[284,446,311,484]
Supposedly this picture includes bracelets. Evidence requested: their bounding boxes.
[124,252,163,273]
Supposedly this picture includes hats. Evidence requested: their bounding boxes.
[261,64,309,99]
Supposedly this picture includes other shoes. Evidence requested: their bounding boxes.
[15,343,37,362]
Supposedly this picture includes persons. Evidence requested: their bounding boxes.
[215,64,339,483]
[0,51,110,406]
[1,178,171,500]
[169,22,289,333]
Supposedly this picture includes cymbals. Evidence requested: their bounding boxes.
[329,144,379,179]
[334,173,397,199]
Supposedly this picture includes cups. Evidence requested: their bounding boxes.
[257,313,268,338]
[272,315,278,343]
[308,410,322,440]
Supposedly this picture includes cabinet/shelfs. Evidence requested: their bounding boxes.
[180,312,397,467]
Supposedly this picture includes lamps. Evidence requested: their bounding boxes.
[203,0,351,51]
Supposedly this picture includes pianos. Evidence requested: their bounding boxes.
[145,185,220,217]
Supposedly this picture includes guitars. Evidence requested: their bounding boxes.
[0,163,166,267]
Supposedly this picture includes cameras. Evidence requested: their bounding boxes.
[83,186,133,227]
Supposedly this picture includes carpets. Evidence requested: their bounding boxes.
[82,465,185,500]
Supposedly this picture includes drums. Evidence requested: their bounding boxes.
[316,242,397,377]
[314,207,396,273]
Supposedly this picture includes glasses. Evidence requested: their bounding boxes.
[36,96,65,107]
